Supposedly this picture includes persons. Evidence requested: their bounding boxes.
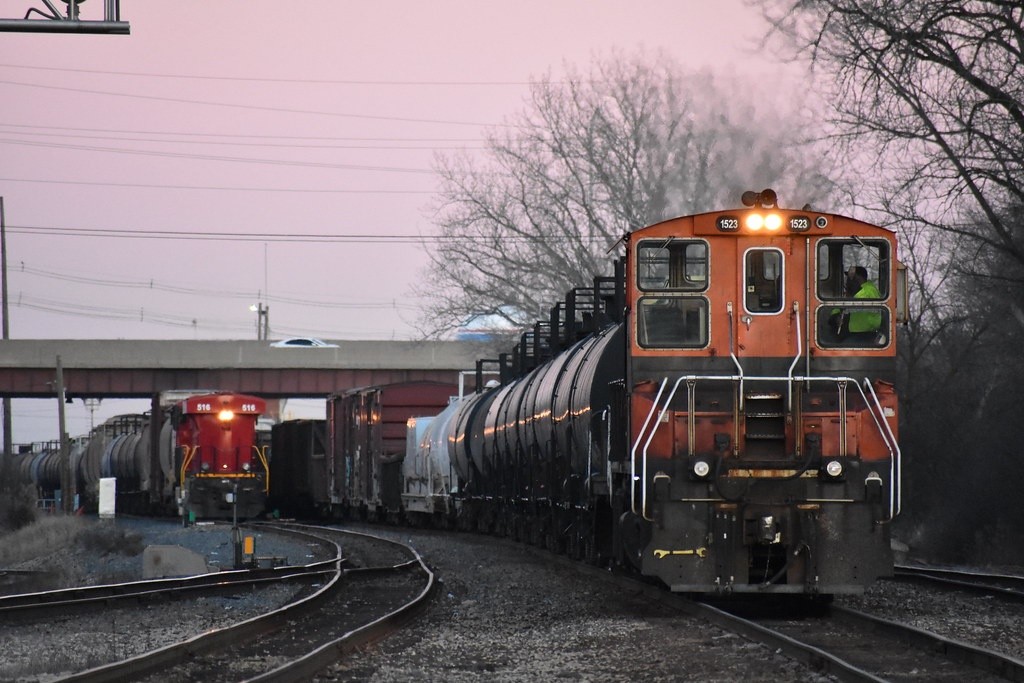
[828,265,883,342]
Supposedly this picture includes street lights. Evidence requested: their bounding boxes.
[250,306,268,341]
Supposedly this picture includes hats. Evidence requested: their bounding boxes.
[844,266,867,280]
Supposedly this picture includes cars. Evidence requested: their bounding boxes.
[269,338,341,348]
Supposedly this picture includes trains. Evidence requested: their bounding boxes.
[1,390,267,523]
[255,187,904,595]
